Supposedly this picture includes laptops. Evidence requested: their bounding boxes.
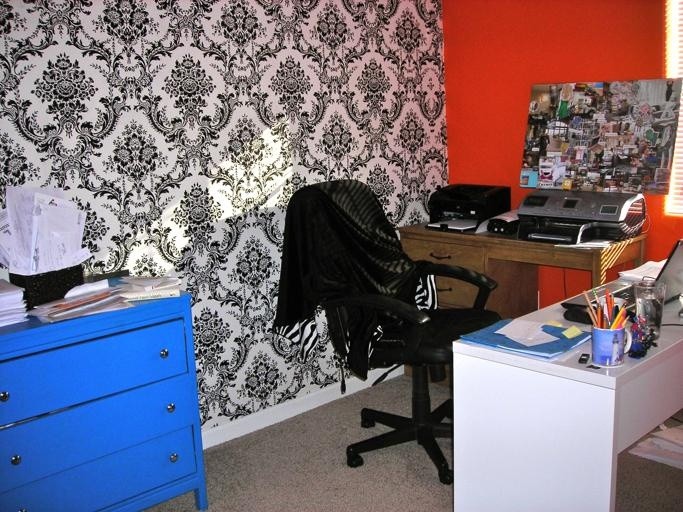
[562,239,683,318]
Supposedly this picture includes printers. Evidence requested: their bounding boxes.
[517,189,647,244]
[425,184,510,233]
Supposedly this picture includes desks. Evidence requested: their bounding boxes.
[398,221,648,377]
[452,258,683,512]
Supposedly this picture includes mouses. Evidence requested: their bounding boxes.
[564,306,593,324]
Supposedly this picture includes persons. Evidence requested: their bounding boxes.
[535,129,551,158]
[521,176,528,185]
[620,122,630,134]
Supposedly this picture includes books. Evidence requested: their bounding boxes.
[0,275,183,328]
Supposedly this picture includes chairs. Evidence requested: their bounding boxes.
[273,180,503,483]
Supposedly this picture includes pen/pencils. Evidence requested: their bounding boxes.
[582,286,632,330]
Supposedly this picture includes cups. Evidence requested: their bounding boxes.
[591,326,633,370]
[632,280,666,341]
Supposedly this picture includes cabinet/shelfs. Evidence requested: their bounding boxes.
[1,276,207,512]
